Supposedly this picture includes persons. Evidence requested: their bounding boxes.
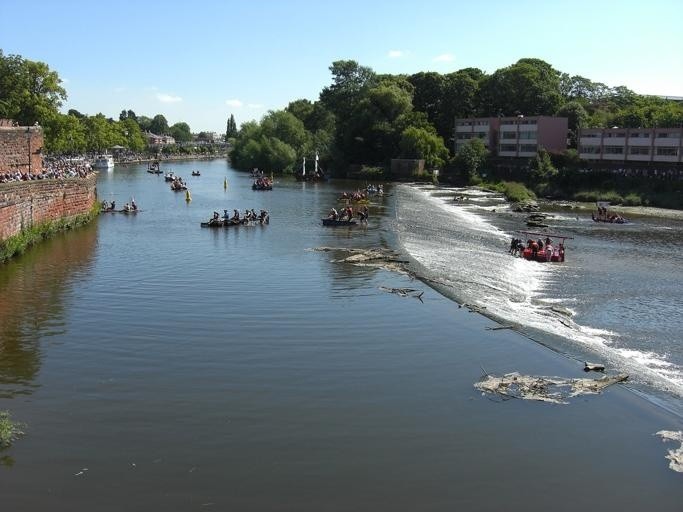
[89,150,220,168]
[255,176,273,187]
[119,202,137,213]
[251,168,262,177]
[454,193,628,260]
[165,172,187,191]
[148,162,162,171]
[315,172,320,184]
[0,152,94,184]
[208,208,269,226]
[101,199,115,211]
[339,183,384,200]
[328,206,369,225]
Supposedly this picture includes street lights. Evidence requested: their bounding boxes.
[28,121,38,174]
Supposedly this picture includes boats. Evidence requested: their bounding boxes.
[192,173,201,176]
[336,187,383,203]
[522,248,563,262]
[147,168,164,174]
[250,173,273,191]
[297,175,328,183]
[164,172,187,191]
[593,216,628,224]
[100,209,142,214]
[199,216,269,229]
[319,217,361,227]
